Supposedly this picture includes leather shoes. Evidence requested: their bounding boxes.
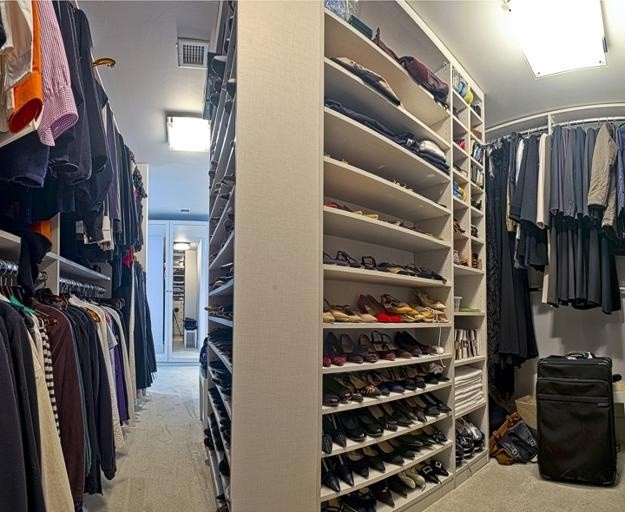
[322,203,451,512]
[201,75,237,512]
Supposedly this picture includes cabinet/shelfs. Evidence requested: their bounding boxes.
[173,250,185,336]
[201,1,490,512]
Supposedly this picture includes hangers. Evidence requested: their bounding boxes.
[0,258,48,298]
[553,115,625,131]
[486,125,549,152]
[59,277,106,298]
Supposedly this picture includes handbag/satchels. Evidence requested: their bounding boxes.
[489,412,537,464]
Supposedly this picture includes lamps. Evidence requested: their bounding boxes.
[505,0,609,80]
[166,116,211,153]
[173,240,191,251]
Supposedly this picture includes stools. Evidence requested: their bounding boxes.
[184,329,197,348]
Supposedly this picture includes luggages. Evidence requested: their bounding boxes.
[536,352,622,486]
